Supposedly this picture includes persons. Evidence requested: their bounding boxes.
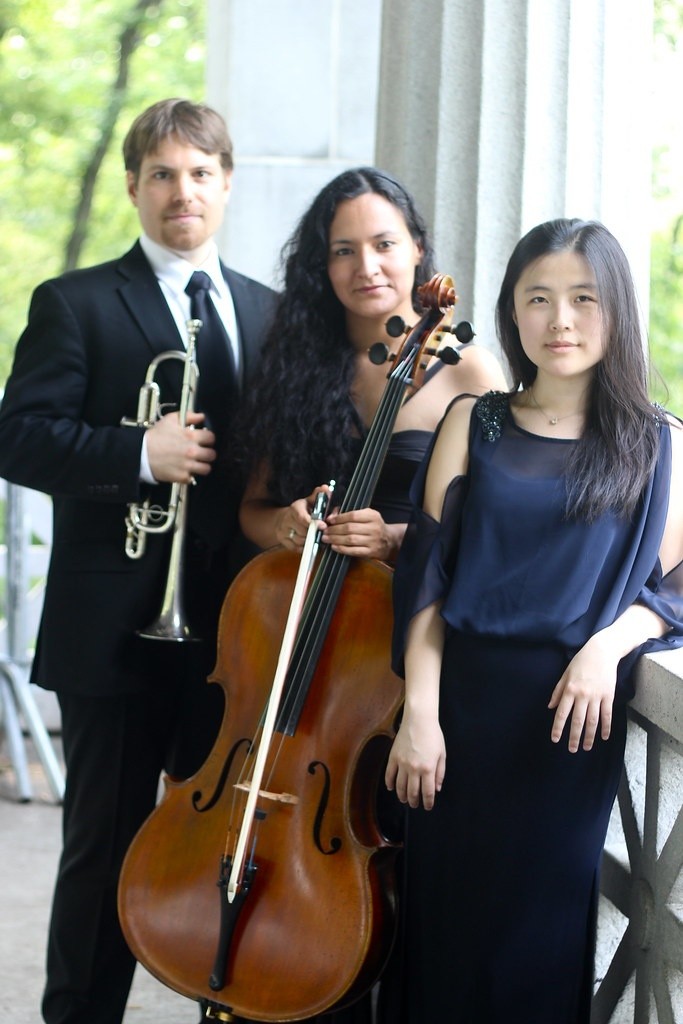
[379,224,683,1024]
[221,169,505,1024]
[0,100,285,1024]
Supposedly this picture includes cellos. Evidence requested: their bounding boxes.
[119,270,476,1024]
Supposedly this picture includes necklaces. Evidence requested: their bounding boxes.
[532,385,589,426]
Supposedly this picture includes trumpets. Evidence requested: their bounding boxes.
[123,322,213,640]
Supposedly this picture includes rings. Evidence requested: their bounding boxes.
[289,528,295,540]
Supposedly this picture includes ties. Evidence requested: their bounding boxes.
[184,271,246,438]
